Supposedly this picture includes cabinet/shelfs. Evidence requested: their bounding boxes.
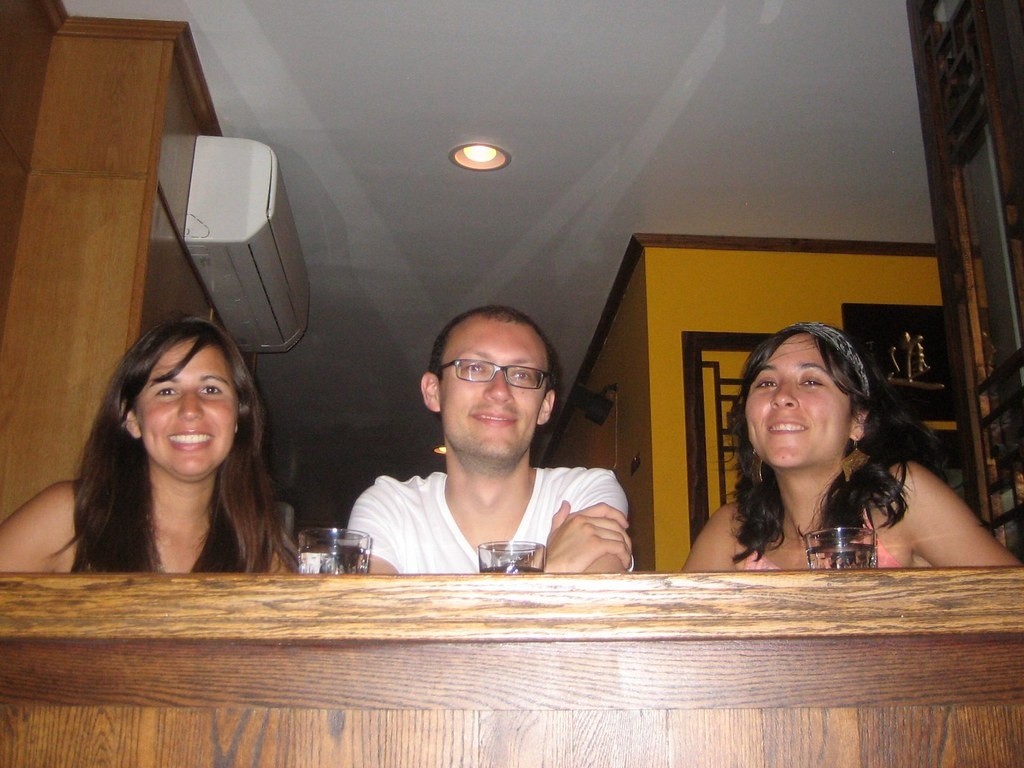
[904,0,1022,554]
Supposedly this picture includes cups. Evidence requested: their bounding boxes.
[296,528,371,577]
[804,526,877,571]
[476,540,547,574]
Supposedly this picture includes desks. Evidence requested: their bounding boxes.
[3,559,1024,766]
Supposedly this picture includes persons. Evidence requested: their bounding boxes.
[673,322,1018,567]
[341,303,639,571]
[0,311,398,577]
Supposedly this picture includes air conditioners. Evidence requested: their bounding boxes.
[183,132,312,357]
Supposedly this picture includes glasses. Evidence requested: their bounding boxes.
[437,359,553,389]
